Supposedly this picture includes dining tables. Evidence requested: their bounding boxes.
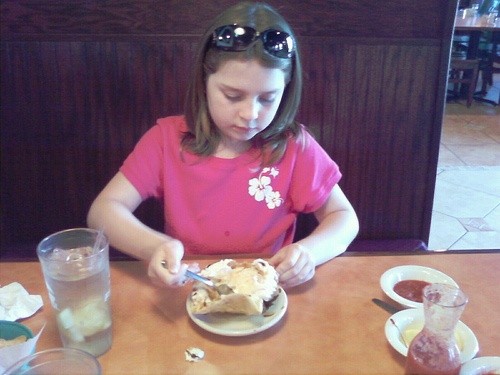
[0,249,500,375]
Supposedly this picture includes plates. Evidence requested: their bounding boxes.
[384,308,479,363]
[186,285,288,336]
[0,320,35,375]
[380,265,460,308]
[459,356,500,375]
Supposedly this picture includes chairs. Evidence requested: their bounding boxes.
[449,59,479,108]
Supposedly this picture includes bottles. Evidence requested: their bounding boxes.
[406,283,468,375]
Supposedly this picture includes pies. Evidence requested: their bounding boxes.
[191,258,278,315]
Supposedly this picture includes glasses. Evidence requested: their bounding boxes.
[202,23,295,60]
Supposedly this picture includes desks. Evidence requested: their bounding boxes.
[454,27,500,107]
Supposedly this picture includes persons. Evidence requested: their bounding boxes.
[86,1,360,290]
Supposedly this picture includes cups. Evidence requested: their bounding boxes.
[2,347,101,375]
[36,227,113,357]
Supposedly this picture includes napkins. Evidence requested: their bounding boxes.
[0,282,44,322]
